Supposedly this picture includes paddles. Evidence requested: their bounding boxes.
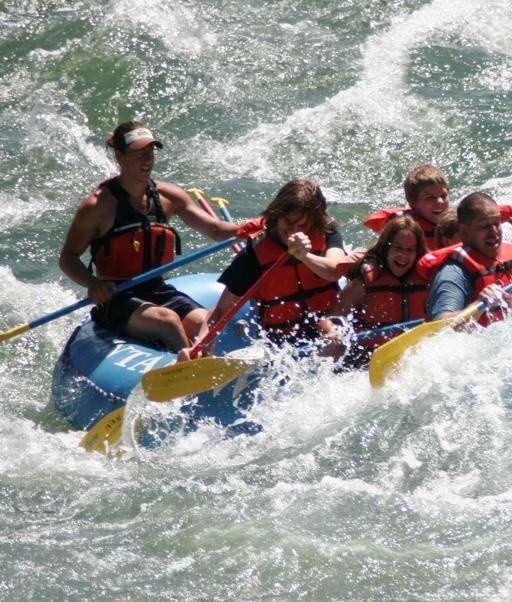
[142,315,426,403]
[77,241,302,457]
[368,282,512,390]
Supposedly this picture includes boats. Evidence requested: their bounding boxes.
[52,271,425,449]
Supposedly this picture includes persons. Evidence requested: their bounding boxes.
[313,215,436,365]
[419,187,511,327]
[59,120,263,353]
[381,164,511,252]
[170,175,351,363]
[430,203,463,249]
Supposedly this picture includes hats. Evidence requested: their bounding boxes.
[120,128,163,152]
